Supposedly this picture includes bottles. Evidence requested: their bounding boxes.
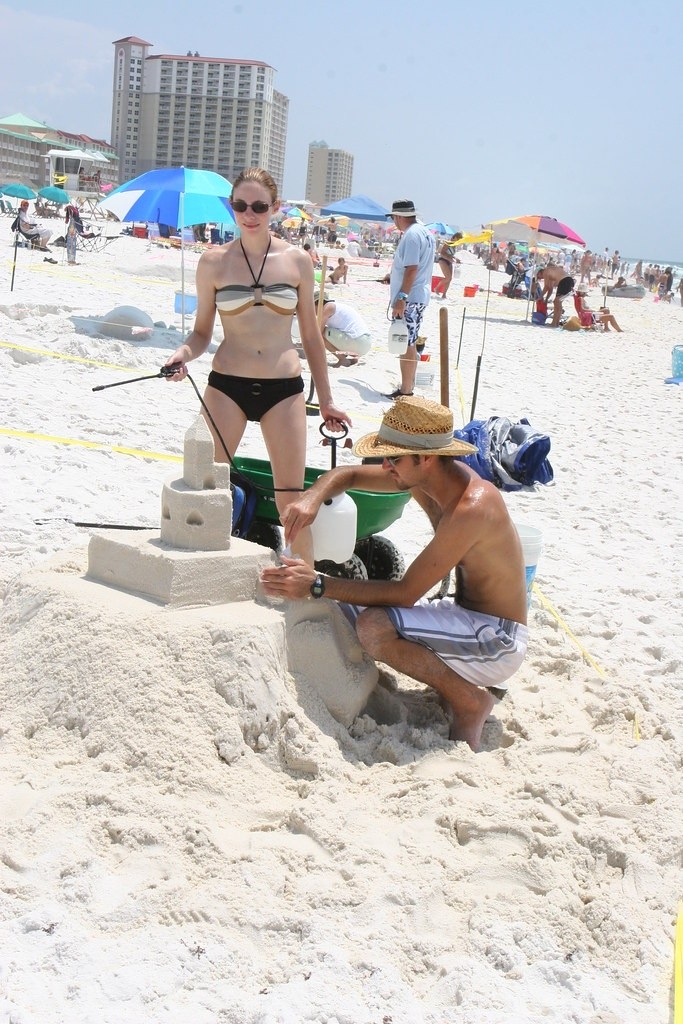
[388,314,408,354]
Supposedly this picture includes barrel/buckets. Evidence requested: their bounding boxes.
[579,312,593,326]
[513,521,543,611]
[174,290,198,314]
[147,223,160,239]
[184,229,194,243]
[134,224,146,238]
[313,490,357,565]
[431,276,445,293]
[464,286,477,297]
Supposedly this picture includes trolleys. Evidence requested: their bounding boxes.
[229,455,412,581]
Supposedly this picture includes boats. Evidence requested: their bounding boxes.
[602,284,646,298]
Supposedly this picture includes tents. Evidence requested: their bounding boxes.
[321,193,395,223]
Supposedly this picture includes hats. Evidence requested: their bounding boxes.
[351,396,479,459]
[534,265,544,283]
[384,200,419,217]
[573,283,590,293]
[313,291,335,305]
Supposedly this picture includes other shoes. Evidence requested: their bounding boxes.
[45,257,58,264]
[381,385,414,401]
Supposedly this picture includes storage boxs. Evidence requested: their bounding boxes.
[420,351,431,361]
[185,230,196,243]
[503,283,522,297]
[464,287,476,297]
[515,523,542,613]
[432,276,445,292]
[148,228,160,237]
[174,289,198,313]
[134,225,147,237]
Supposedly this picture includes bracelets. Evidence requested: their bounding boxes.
[397,292,408,300]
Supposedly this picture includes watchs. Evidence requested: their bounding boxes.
[310,574,325,599]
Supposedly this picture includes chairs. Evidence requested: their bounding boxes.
[507,258,609,331]
[0,174,234,254]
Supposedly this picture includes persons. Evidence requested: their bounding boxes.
[271,215,402,283]
[18,200,52,251]
[261,393,526,751]
[435,233,683,301]
[307,289,373,369]
[78,166,102,183]
[378,198,434,399]
[575,284,623,333]
[532,264,575,327]
[165,168,354,568]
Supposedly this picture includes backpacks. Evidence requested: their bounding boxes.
[224,470,256,538]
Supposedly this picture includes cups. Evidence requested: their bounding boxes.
[671,344,683,378]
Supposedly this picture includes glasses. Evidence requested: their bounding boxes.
[228,200,275,214]
[22,204,29,207]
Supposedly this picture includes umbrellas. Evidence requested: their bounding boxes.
[278,205,313,221]
[316,212,351,223]
[1,183,37,200]
[424,221,457,235]
[38,185,71,204]
[481,216,585,324]
[97,165,242,340]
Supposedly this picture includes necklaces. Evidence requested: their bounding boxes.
[238,235,271,305]
